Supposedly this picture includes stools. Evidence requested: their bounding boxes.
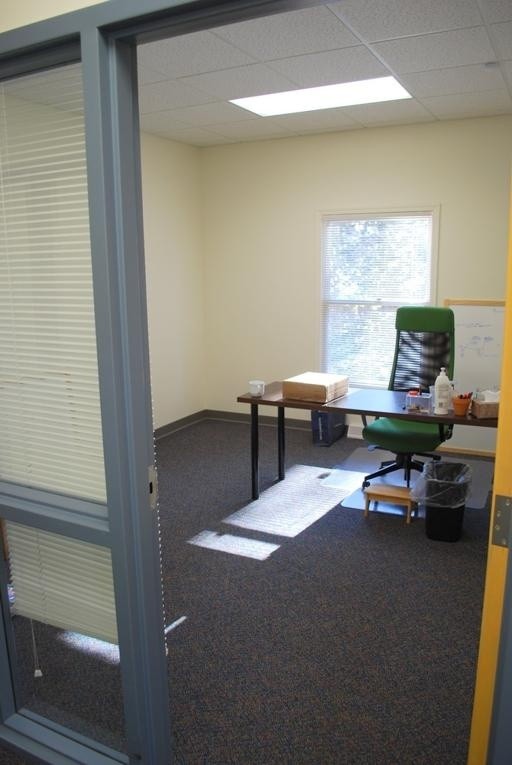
[363,483,418,524]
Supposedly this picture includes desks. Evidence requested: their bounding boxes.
[236,380,498,501]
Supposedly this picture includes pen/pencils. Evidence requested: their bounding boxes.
[457,392,473,399]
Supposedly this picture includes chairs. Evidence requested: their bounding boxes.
[362,306,455,488]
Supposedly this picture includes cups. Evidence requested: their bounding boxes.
[249,381,264,396]
[452,396,470,416]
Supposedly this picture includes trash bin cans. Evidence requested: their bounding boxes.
[424,460,471,542]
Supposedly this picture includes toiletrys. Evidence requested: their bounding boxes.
[435,367,451,415]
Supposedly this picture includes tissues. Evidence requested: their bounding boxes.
[472,390,499,419]
